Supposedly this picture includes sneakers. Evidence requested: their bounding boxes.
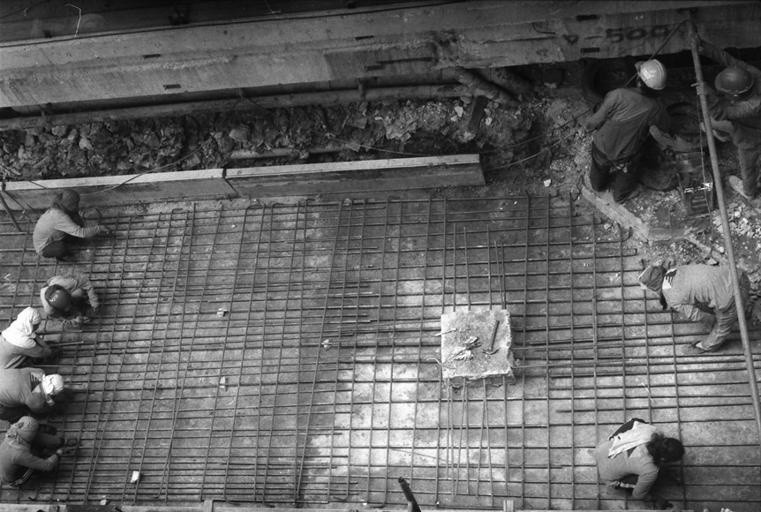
[729,175,755,201]
[681,340,710,355]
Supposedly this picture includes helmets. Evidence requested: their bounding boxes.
[49,289,72,313]
[713,65,754,97]
[634,58,668,91]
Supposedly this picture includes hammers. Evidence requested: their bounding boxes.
[482,320,500,355]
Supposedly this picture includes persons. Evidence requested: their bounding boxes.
[591,416,685,511]
[39,266,102,327]
[687,28,761,204]
[583,58,670,204]
[30,188,111,263]
[0,415,65,490]
[0,307,53,369]
[0,367,65,424]
[636,262,752,354]
